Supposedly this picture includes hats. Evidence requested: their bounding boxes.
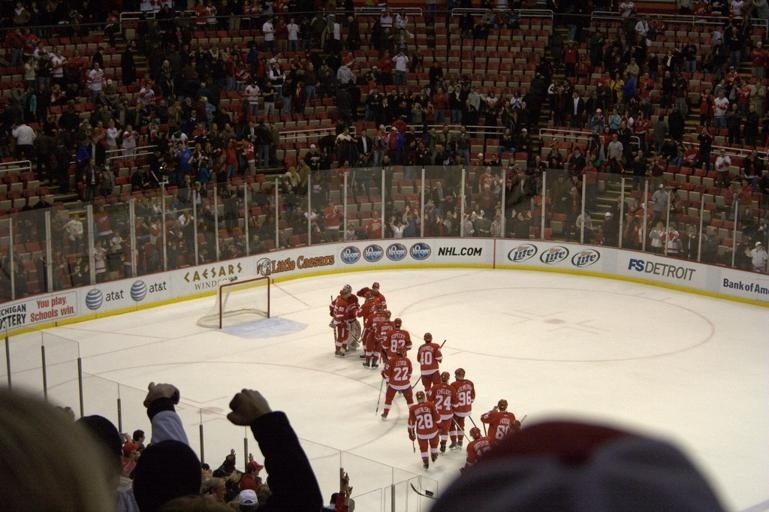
[238,489,260,506]
[245,459,264,474]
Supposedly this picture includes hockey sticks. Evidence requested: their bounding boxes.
[411,340,445,388]
[483,422,489,438]
[452,417,470,444]
[412,426,416,453]
[376,378,385,414]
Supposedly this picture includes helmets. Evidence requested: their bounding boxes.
[339,281,391,320]
[396,345,406,356]
[393,318,402,328]
[423,332,433,342]
[468,427,481,440]
[497,399,509,411]
[439,372,451,383]
[455,367,466,378]
[415,390,424,400]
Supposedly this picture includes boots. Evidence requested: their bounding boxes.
[336,344,380,368]
[380,410,389,418]
[422,440,463,469]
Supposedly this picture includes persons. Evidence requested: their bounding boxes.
[329,284,520,470]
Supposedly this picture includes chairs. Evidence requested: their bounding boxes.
[1,0,769,302]
